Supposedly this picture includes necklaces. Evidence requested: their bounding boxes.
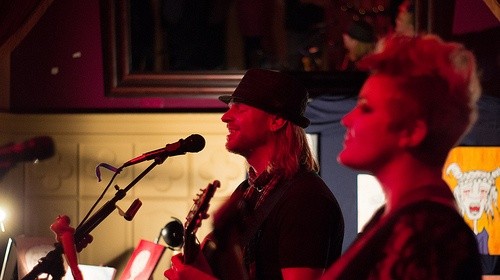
[255,186,265,192]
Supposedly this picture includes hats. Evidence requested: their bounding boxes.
[217,65,311,129]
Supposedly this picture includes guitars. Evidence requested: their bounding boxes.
[183,180,221,267]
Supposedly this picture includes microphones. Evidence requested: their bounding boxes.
[123,134,205,169]
[1,135,55,171]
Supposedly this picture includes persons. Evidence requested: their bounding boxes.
[323,31,482,280]
[164,65,345,280]
